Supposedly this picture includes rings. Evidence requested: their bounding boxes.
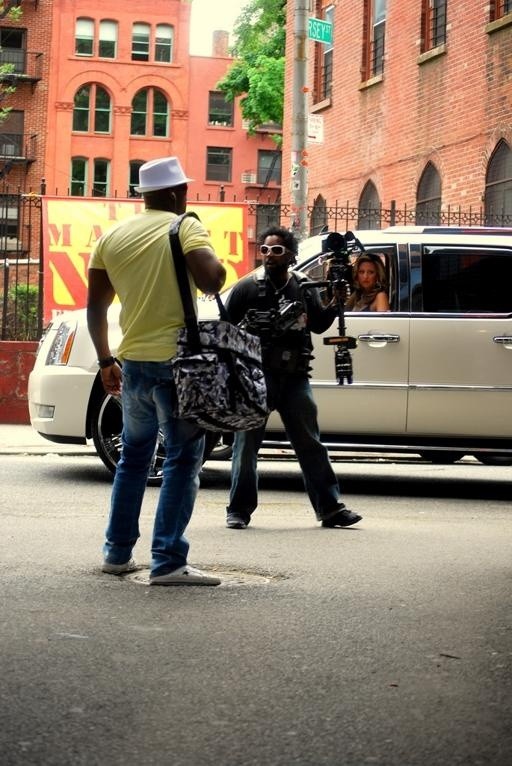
[112,385,116,388]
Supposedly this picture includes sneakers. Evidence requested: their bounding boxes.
[100,556,138,575]
[149,563,222,588]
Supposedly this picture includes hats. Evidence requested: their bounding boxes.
[133,155,195,195]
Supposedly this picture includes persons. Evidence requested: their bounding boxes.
[220,227,362,527]
[87,156,228,587]
[430,255,503,313]
[346,253,391,313]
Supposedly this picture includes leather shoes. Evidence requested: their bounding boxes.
[226,511,247,530]
[320,508,364,529]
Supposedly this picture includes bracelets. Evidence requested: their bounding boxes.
[95,356,115,368]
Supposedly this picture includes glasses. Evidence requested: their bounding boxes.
[259,243,295,258]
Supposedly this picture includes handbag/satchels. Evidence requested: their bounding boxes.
[172,318,267,435]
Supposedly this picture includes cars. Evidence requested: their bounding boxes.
[27,224,512,488]
